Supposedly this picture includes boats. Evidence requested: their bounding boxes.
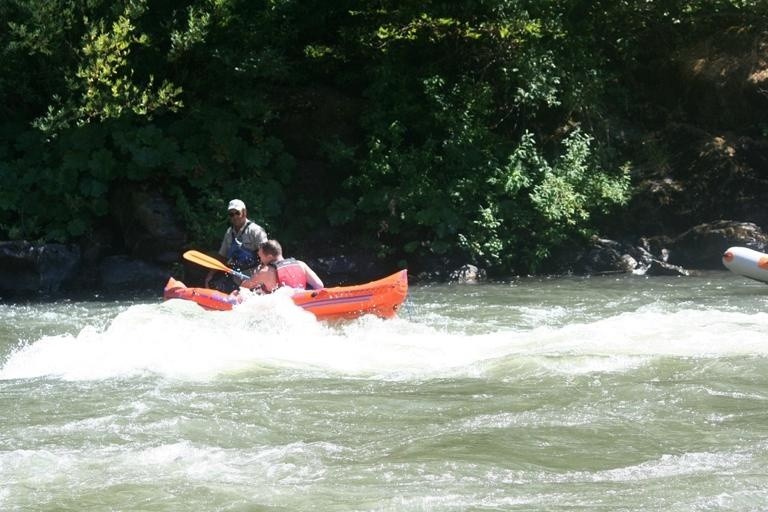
[722,246,768,284]
[163,268,408,321]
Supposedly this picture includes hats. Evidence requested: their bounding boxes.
[228,199,246,211]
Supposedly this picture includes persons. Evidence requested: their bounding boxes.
[203,197,269,295]
[237,240,325,297]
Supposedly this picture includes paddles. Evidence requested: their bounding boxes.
[183,249,251,281]
